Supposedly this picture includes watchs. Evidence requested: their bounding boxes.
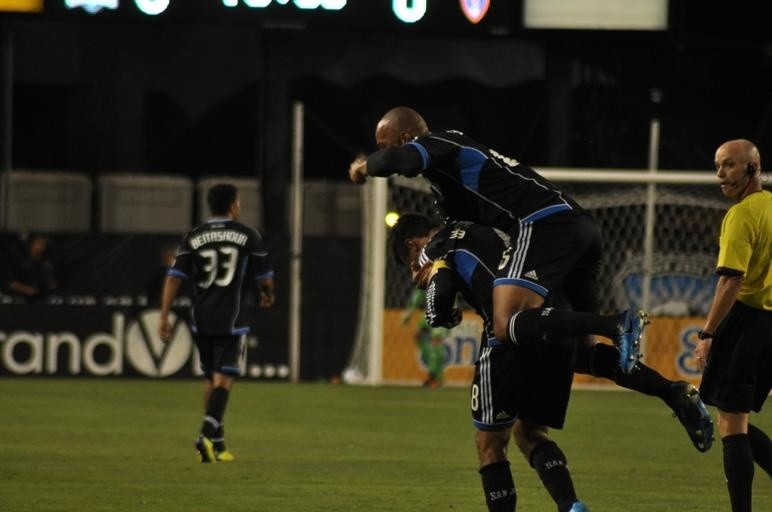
[697,331,713,340]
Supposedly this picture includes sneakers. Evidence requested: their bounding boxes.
[195,433,216,464]
[213,449,235,462]
[671,379,715,454]
[423,372,444,389]
[614,308,652,374]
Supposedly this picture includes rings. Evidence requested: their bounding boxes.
[695,356,700,359]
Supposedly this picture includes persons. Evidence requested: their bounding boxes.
[400,284,450,388]
[5,233,58,304]
[144,235,184,299]
[693,135,772,512]
[157,179,276,464]
[392,209,589,512]
[345,105,717,456]
